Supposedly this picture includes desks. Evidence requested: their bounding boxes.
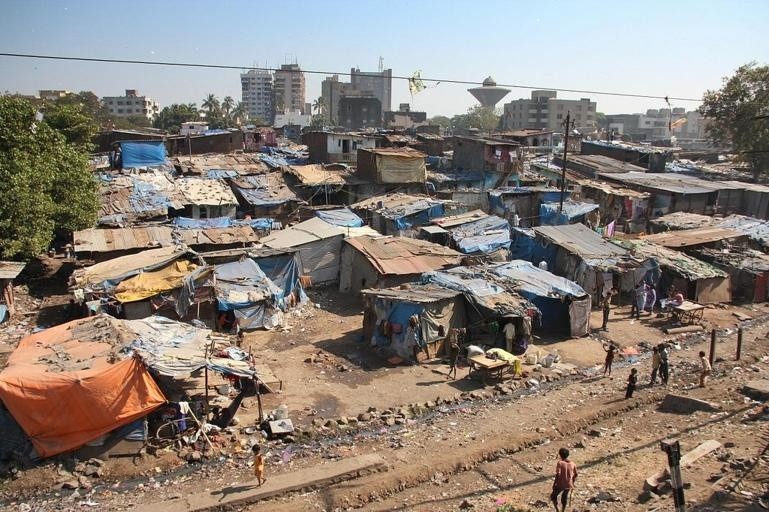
[469,354,508,387]
[668,300,705,324]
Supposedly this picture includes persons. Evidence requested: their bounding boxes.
[551,448,578,512]
[503,319,516,352]
[513,211,522,227]
[251,444,266,488]
[539,257,548,270]
[66,241,73,259]
[597,128,615,141]
[447,343,459,380]
[220,313,231,330]
[602,281,684,332]
[603,343,712,399]
